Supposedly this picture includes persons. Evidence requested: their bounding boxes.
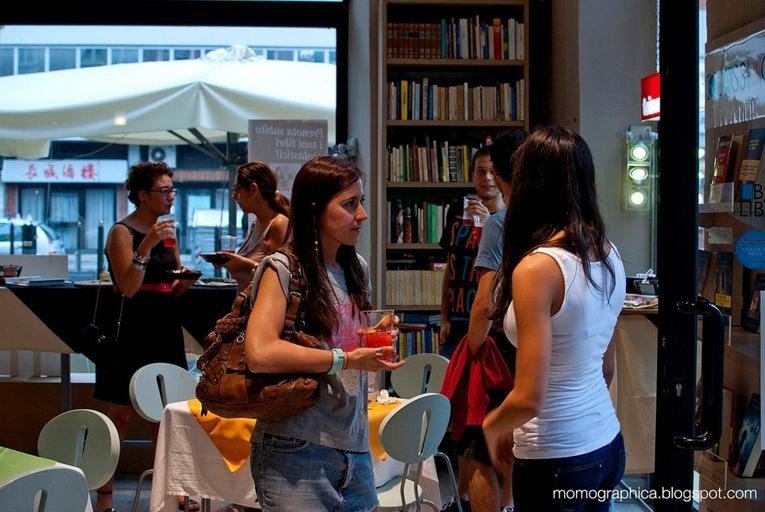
[481,123,626,512]
[457,127,530,512]
[244,154,406,512]
[435,144,517,512]
[89,161,203,511]
[211,160,294,312]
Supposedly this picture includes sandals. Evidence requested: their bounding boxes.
[178,497,200,511]
[93,490,116,512]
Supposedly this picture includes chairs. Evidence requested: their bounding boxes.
[0,466,89,512]
[390,352,452,413]
[372,391,463,512]
[37,407,121,512]
[0,254,69,379]
[128,362,198,511]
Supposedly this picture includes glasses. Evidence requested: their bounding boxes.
[145,187,178,197]
[232,184,251,193]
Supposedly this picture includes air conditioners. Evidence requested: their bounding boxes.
[148,142,178,172]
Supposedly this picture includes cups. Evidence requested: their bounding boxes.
[0,262,22,284]
[157,215,178,249]
[472,214,490,243]
[463,196,482,225]
[220,235,236,256]
[359,310,395,360]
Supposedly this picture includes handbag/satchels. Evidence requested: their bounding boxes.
[195,249,325,422]
[82,322,120,354]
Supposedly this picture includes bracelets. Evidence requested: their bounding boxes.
[130,250,151,272]
[345,352,349,370]
[327,348,344,376]
[251,261,259,276]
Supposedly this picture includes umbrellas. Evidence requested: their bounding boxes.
[0,45,338,233]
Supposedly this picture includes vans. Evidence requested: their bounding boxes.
[0,217,65,256]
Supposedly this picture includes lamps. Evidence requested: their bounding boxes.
[621,124,658,214]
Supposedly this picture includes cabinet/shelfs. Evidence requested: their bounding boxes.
[368,0,531,454]
[703,1,765,469]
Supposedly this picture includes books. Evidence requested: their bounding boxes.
[383,14,530,395]
[707,126,764,479]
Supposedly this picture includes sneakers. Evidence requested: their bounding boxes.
[440,494,472,511]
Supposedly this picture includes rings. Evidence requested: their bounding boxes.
[376,348,384,356]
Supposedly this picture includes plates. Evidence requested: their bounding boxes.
[200,253,233,264]
[167,270,202,280]
